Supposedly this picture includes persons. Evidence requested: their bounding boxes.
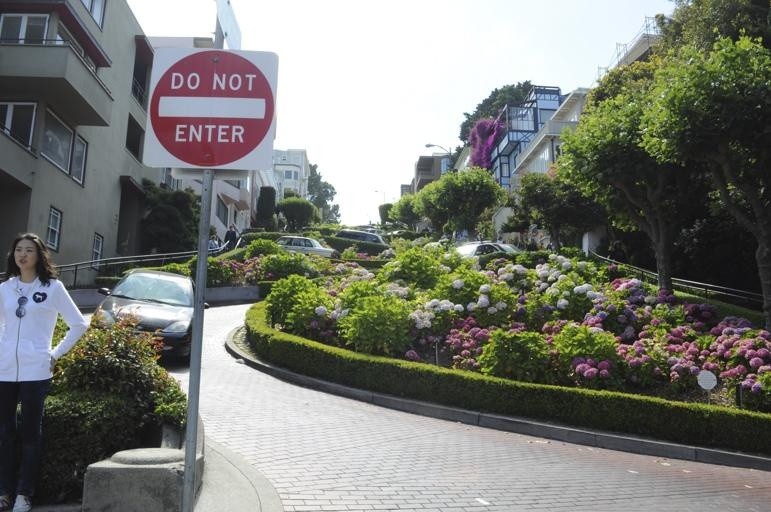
[496,237,504,244]
[456,231,464,240]
[0,233,88,511]
[208,235,219,256]
[548,239,558,252]
[224,225,236,252]
[527,238,538,251]
[284,225,288,232]
[611,241,630,265]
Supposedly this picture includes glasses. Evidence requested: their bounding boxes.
[16,296,27,317]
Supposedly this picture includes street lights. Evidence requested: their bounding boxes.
[356,224,374,228]
[373,189,385,203]
[424,143,452,173]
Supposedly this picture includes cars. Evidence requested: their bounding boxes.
[243,235,342,260]
[362,228,384,235]
[386,230,416,236]
[335,229,391,244]
[91,268,211,360]
[444,239,521,267]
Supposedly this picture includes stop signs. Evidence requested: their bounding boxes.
[143,51,276,172]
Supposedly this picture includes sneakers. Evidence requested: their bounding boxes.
[0,494,33,512]
